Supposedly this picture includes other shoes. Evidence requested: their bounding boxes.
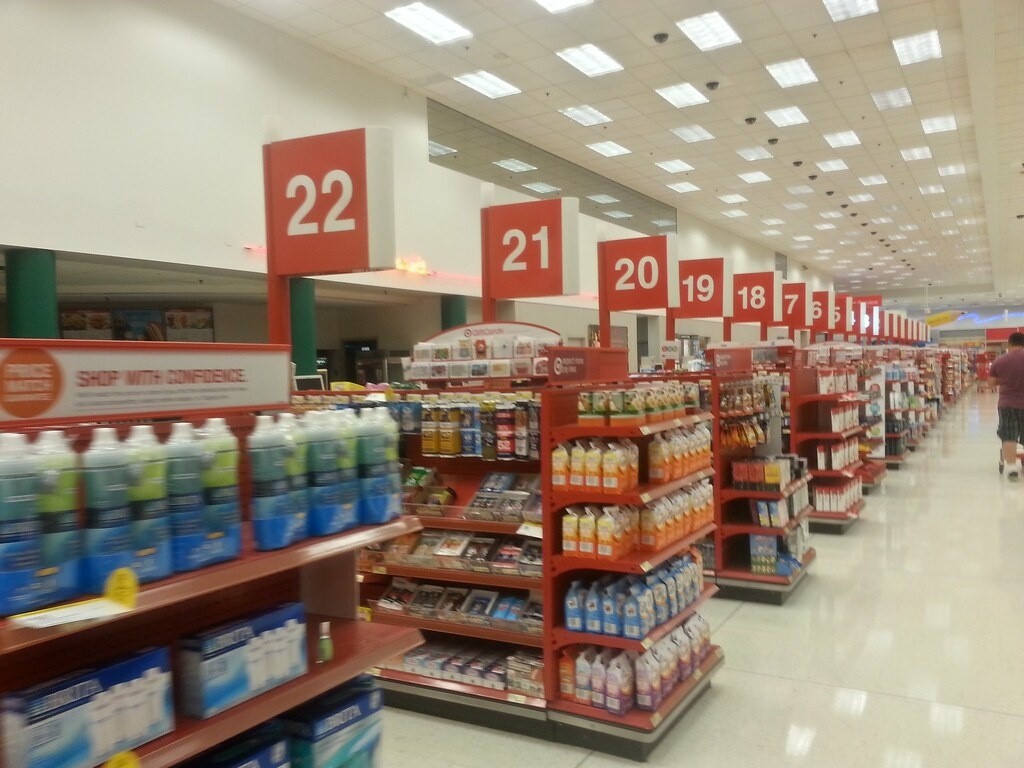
[1008,472,1018,482]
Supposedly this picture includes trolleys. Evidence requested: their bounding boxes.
[975,377,998,393]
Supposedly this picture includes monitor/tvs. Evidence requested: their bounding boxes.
[295,369,328,394]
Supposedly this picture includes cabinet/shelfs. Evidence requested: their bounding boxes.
[630,348,816,606]
[0,337,426,768]
[749,349,866,535]
[302,348,728,763]
[858,338,976,492]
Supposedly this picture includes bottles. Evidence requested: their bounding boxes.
[318,622,334,661]
[246,407,401,550]
[0,416,238,618]
[247,619,304,689]
[88,668,164,757]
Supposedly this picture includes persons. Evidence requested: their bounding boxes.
[987,332,1024,481]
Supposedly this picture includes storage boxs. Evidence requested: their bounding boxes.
[831,437,859,470]
[368,575,544,635]
[751,514,809,576]
[756,501,771,527]
[407,643,545,694]
[0,645,176,768]
[812,474,862,513]
[177,601,310,719]
[273,674,383,743]
[817,445,828,470]
[733,454,808,491]
[465,470,545,524]
[789,483,809,517]
[367,529,544,580]
[769,499,789,528]
[832,401,860,433]
[398,457,457,515]
[292,392,540,462]
[819,367,858,395]
[193,724,290,768]
